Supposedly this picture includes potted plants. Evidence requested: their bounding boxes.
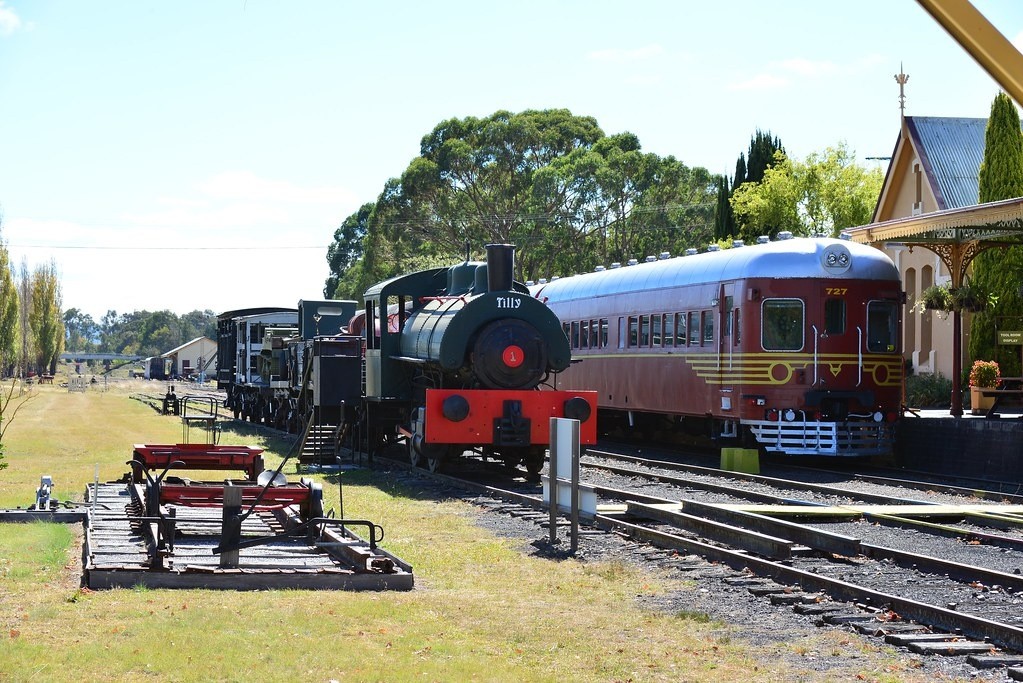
[969,360,1002,415]
[909,273,989,320]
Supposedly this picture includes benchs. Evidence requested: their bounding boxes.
[975,390,1023,418]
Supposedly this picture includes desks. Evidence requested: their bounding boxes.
[991,378,1023,390]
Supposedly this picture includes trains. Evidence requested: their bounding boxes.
[215,242,598,475]
[356,230,907,457]
